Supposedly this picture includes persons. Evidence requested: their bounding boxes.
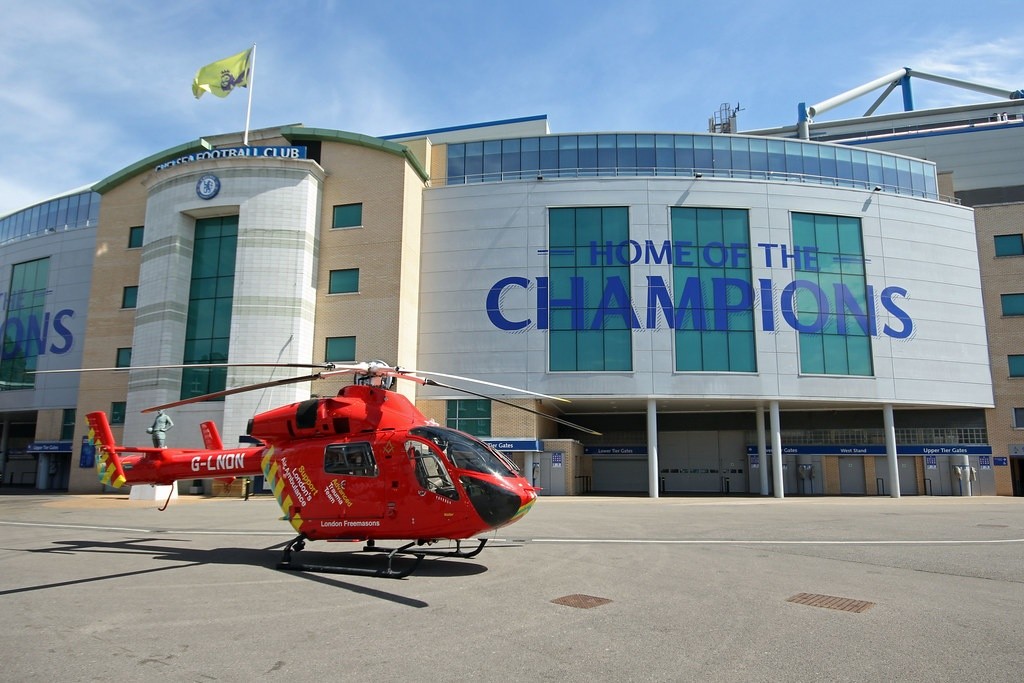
[146,410,174,448]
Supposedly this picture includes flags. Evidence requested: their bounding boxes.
[192,45,256,100]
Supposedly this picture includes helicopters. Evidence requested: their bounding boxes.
[25,357,605,581]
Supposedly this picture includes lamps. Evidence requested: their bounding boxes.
[695,172,702,181]
[537,175,544,181]
[872,186,881,193]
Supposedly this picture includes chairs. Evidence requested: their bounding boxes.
[421,455,467,500]
